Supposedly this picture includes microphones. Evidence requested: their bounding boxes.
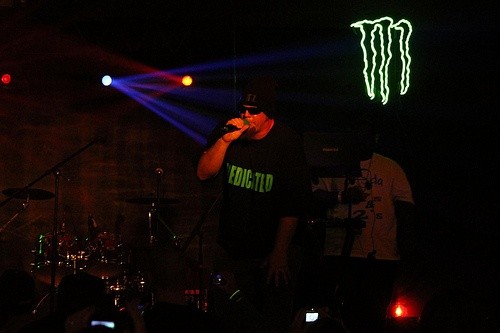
[90,213,101,232]
[221,120,250,132]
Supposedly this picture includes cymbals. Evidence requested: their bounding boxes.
[1,186,56,201]
[126,197,180,205]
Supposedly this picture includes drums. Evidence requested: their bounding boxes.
[29,230,83,289]
[80,231,131,279]
[27,275,144,333]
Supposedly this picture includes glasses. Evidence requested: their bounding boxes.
[238,106,262,115]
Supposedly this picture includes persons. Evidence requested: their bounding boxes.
[197,76,312,333]
[324,117,414,333]
[0,267,111,333]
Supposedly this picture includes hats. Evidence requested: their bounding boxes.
[240,76,279,114]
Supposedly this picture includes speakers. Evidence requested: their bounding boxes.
[291,308,351,333]
